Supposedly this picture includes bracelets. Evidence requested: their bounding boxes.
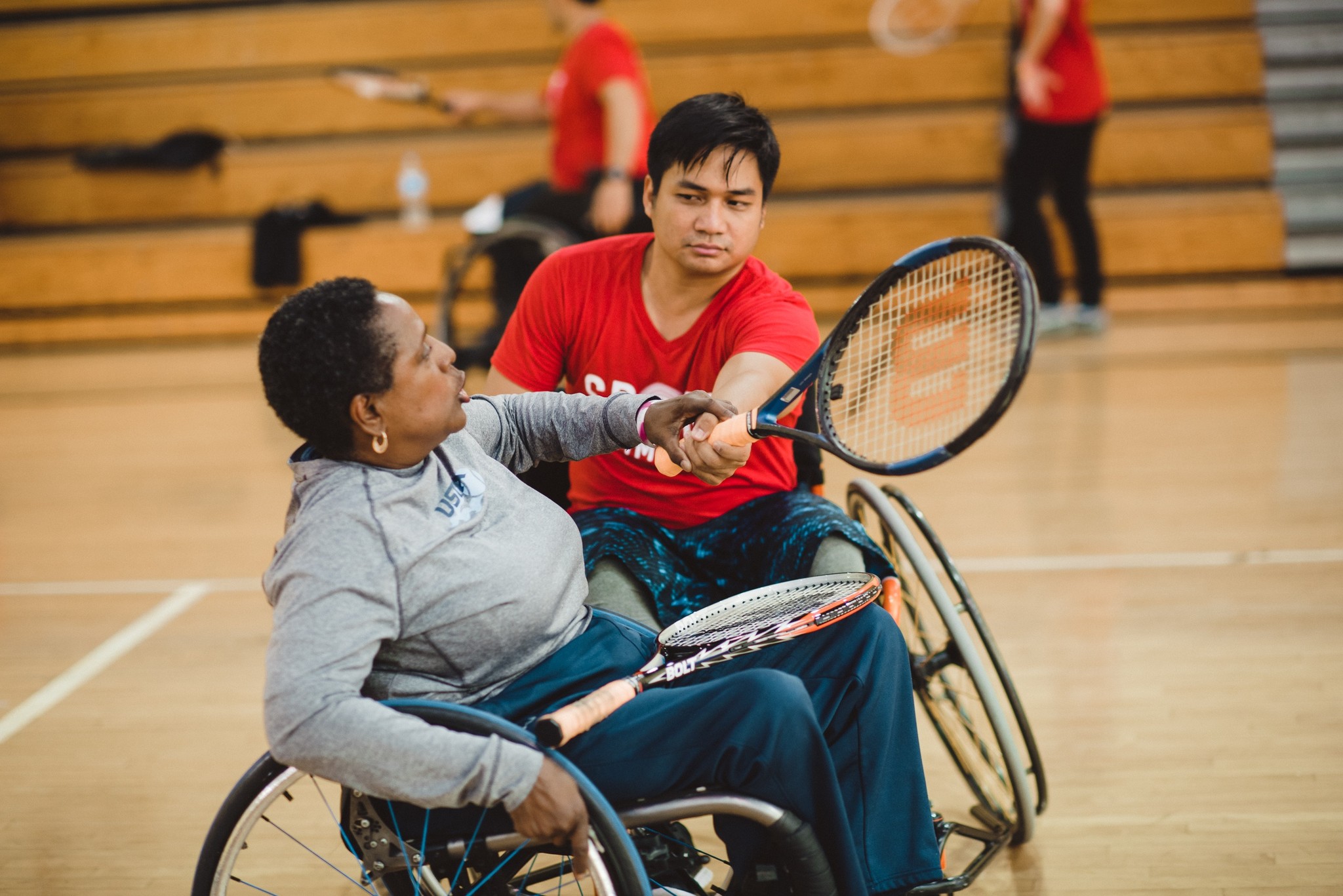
[602,167,631,182]
[636,399,664,448]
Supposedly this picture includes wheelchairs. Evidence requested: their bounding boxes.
[187,377,1053,896]
[436,174,650,374]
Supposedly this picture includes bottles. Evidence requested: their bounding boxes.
[397,151,428,231]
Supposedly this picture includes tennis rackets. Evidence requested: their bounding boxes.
[532,571,884,750]
[653,232,1040,477]
[321,61,471,119]
[867,0,980,60]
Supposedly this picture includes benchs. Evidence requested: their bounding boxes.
[0,0,1343,347]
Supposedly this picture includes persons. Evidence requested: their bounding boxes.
[545,0,652,240]
[259,275,942,896]
[996,0,1107,334]
[484,91,898,896]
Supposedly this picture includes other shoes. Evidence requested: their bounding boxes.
[1038,302,1100,331]
[636,821,714,896]
